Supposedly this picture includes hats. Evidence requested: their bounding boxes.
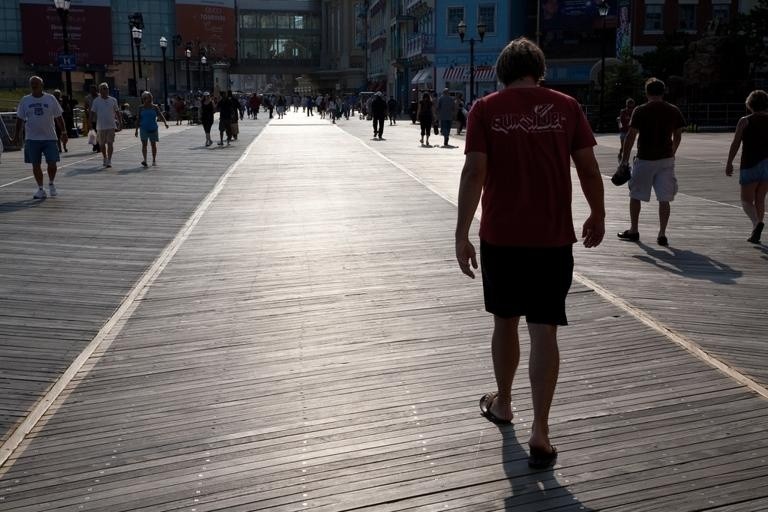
[374,91,383,97]
[203,91,210,96]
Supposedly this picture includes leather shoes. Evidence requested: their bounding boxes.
[747,222,765,244]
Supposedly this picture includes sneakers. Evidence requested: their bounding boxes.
[617,229,642,242]
[240,109,416,124]
[379,137,386,141]
[48,181,58,196]
[374,133,377,137]
[176,121,238,147]
[419,130,463,146]
[33,188,48,199]
[656,236,668,246]
[102,157,156,167]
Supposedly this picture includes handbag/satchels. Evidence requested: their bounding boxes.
[433,124,439,135]
[610,164,631,186]
[87,128,98,145]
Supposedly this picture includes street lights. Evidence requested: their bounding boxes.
[183,46,193,93]
[200,54,208,92]
[598,4,610,116]
[54,1,79,140]
[131,24,145,105]
[457,20,485,104]
[159,34,170,121]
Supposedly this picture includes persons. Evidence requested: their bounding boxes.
[726,89,768,242]
[618,97,636,160]
[302,93,356,123]
[363,91,402,140]
[86,82,121,167]
[410,88,488,147]
[617,77,687,245]
[136,91,168,166]
[2,76,70,198]
[176,91,243,145]
[120,103,137,127]
[455,37,605,470]
[239,92,299,119]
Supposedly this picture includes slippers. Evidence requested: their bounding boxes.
[478,390,513,425]
[527,440,557,470]
